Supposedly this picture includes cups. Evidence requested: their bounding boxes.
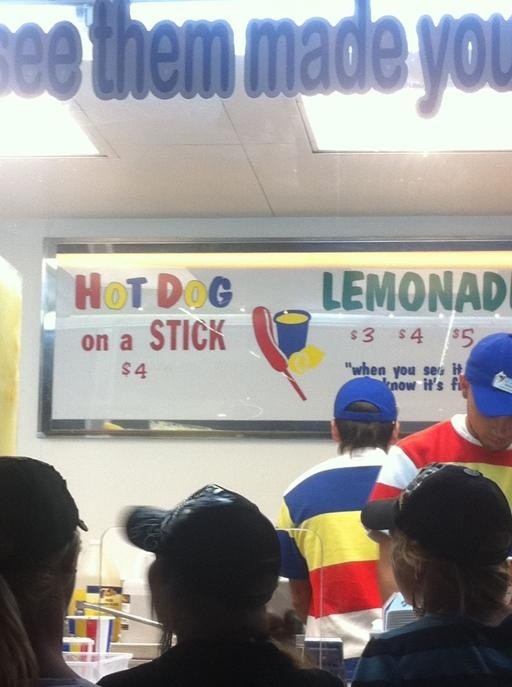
[273,308,311,359]
[62,636,95,663]
[67,615,115,651]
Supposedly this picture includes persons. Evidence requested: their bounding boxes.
[0,456,96,686]
[352,463,512,687]
[351,333,512,605]
[278,376,399,683]
[96,485,346,686]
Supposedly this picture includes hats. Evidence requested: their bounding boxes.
[465,333,511,417]
[1,457,88,568]
[126,484,281,607]
[335,376,397,424]
[362,462,511,568]
